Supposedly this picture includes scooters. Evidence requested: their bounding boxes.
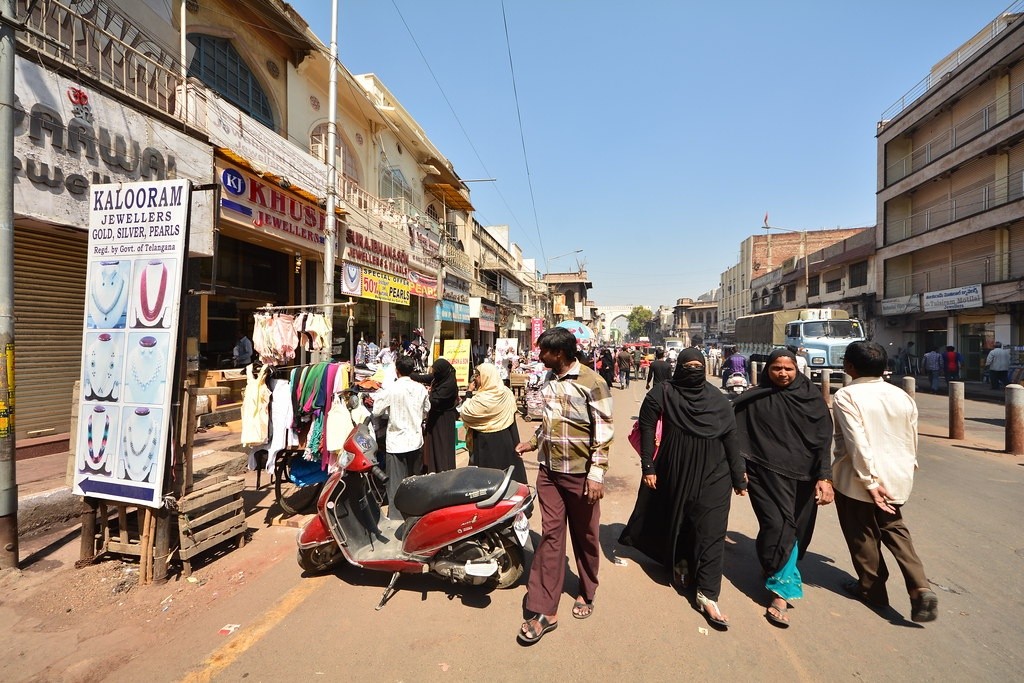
[294,394,538,611]
[721,364,748,397]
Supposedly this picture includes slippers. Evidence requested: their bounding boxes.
[572,601,594,618]
[519,613,558,642]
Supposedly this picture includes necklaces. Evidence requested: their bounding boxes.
[79,259,176,478]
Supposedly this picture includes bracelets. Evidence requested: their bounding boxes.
[824,478,833,484]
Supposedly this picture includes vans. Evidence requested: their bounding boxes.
[622,339,685,373]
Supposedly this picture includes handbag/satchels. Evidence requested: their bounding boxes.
[628,418,662,461]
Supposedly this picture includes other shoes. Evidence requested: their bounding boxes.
[765,601,789,626]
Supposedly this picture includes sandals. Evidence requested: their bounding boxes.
[691,589,728,625]
[673,559,690,589]
[840,578,872,602]
[910,589,938,622]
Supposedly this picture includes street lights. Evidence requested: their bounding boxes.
[546,249,584,318]
[762,226,809,309]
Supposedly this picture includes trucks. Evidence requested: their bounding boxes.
[734,307,872,383]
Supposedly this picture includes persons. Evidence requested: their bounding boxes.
[375,339,530,518]
[984,341,1013,391]
[729,348,836,628]
[898,341,915,374]
[596,339,945,390]
[616,346,747,628]
[515,325,617,645]
[920,345,945,394]
[942,345,965,395]
[231,330,253,368]
[833,340,939,623]
